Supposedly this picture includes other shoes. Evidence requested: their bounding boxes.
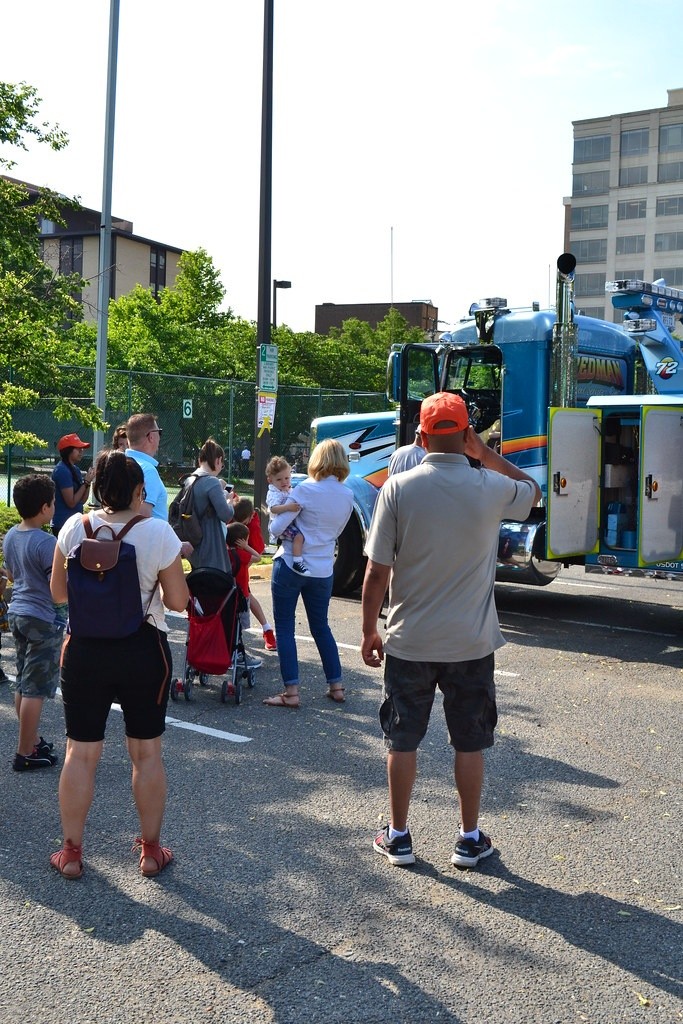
[0,668,8,682]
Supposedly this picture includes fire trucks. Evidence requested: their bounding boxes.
[268,252,683,585]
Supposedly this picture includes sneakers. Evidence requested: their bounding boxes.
[235,653,263,669]
[262,630,276,651]
[13,752,56,771]
[372,825,415,865]
[34,737,53,752]
[292,562,311,576]
[451,823,493,867]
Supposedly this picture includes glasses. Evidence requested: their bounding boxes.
[146,429,163,436]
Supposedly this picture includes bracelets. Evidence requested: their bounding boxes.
[81,479,90,489]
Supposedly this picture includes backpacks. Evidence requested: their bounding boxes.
[67,515,168,704]
[168,474,212,546]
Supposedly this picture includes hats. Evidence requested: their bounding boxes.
[420,391,469,435]
[58,434,91,449]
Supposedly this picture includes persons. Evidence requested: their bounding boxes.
[50,433,97,540]
[49,451,190,879]
[241,445,251,478]
[263,439,354,707]
[182,439,278,669]
[2,474,69,772]
[360,391,543,867]
[111,412,169,522]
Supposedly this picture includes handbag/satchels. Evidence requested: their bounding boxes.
[188,598,230,675]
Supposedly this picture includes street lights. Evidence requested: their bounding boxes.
[273,279,291,330]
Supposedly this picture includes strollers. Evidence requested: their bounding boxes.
[170,548,256,705]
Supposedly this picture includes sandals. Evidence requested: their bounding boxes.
[49,840,85,879]
[326,688,346,703]
[130,838,173,876]
[263,692,299,708]
